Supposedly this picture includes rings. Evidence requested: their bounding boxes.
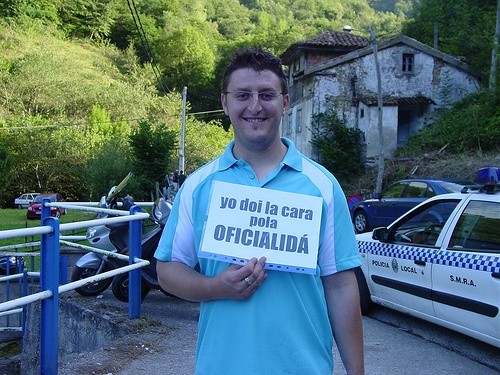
[245,277,252,285]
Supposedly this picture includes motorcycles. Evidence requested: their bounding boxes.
[111,175,180,302]
[71,185,151,297]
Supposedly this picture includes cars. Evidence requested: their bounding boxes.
[14,193,41,209]
[354,184,500,349]
[349,167,500,235]
[27,193,68,219]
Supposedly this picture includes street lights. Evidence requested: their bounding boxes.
[342,25,384,199]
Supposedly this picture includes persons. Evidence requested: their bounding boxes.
[178,171,186,187]
[173,170,178,183]
[153,49,364,375]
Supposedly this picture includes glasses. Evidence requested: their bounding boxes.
[223,91,284,103]
